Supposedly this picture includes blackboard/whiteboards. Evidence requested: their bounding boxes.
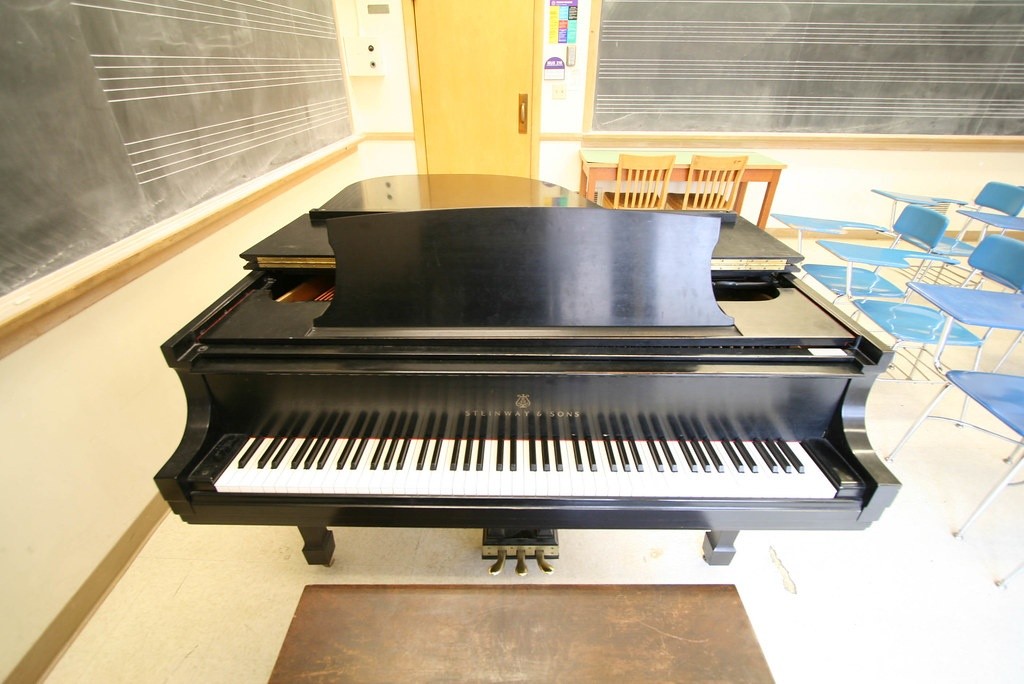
[0,0,367,341]
[580,0,1024,154]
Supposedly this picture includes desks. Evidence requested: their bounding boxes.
[871,190,968,239]
[953,210,1024,252]
[815,239,962,301]
[905,280,1024,447]
[769,213,888,264]
[577,148,788,232]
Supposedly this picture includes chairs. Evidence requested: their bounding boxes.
[922,181,1024,288]
[883,370,1024,536]
[666,154,749,212]
[601,153,676,211]
[850,232,1024,428]
[801,204,949,324]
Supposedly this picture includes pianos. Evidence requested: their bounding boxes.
[152,173,903,577]
